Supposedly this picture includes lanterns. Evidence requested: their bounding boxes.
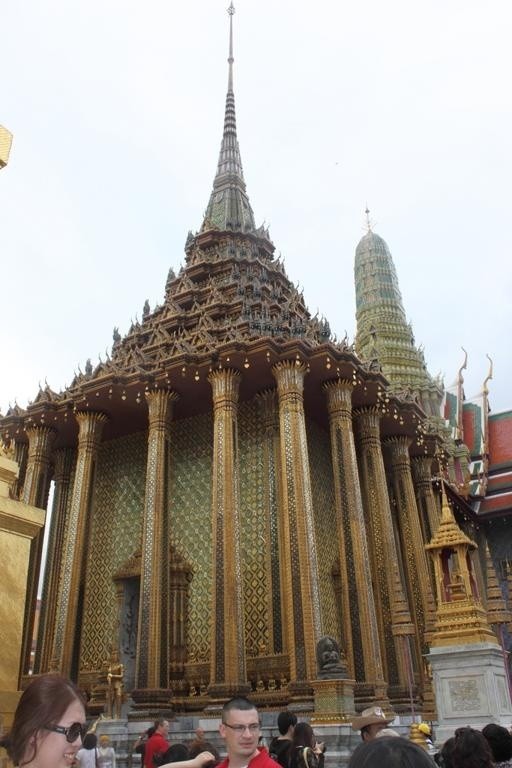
[0,121,15,172]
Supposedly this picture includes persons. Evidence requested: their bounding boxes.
[349,736,439,768]
[418,723,434,749]
[133,720,220,768]
[103,646,125,720]
[352,706,395,744]
[434,724,511,768]
[211,697,325,768]
[0,673,88,768]
[75,734,115,768]
[322,638,348,672]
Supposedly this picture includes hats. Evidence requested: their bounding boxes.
[351,707,393,732]
[418,724,434,736]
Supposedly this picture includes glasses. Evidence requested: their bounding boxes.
[42,722,90,742]
[221,722,260,735]
